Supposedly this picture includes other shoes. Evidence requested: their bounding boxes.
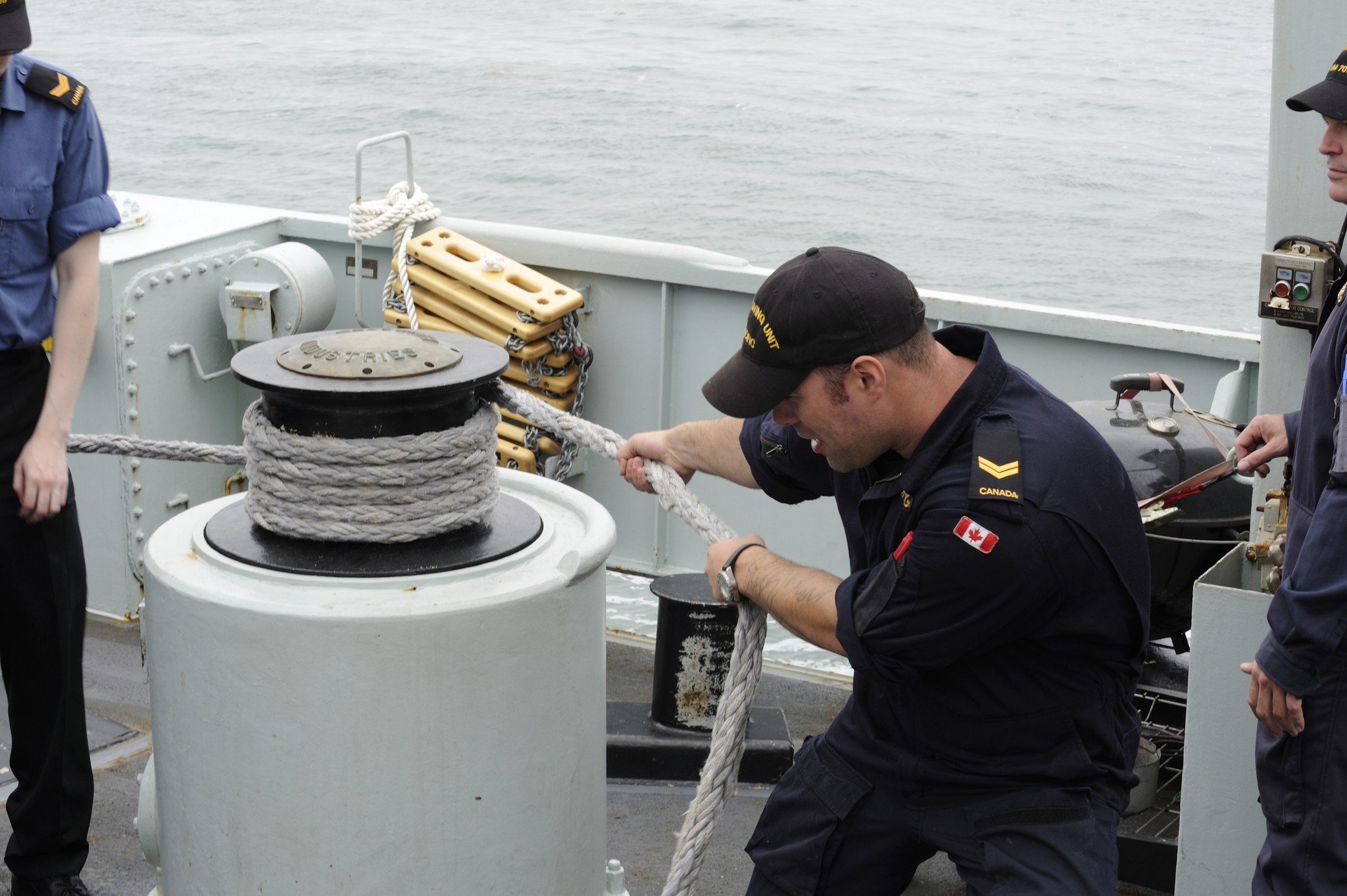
[11,871,92,896]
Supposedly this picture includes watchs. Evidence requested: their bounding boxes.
[716,543,765,605]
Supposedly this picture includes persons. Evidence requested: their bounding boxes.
[0,0,120,896]
[617,247,1150,896]
[1232,50,1347,896]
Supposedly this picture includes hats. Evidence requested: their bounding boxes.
[701,246,925,419]
[1286,46,1347,121]
[0,0,32,51]
